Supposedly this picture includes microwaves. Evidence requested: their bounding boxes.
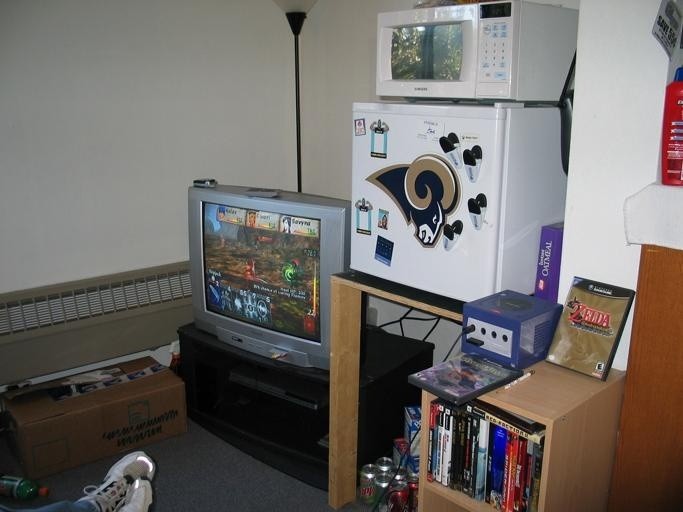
[376,0,579,105]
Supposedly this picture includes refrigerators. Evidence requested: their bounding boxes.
[348,100,572,307]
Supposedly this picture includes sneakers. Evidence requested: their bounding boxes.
[79,451,158,511]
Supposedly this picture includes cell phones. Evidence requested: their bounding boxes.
[193,179,217,187]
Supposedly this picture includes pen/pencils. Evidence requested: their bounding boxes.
[496,370,535,394]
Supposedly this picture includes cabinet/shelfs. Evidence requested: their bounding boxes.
[323,271,629,510]
[177,323,436,496]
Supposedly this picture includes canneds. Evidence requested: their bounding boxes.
[359,456,418,512]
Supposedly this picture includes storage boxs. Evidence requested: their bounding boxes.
[0,355,189,483]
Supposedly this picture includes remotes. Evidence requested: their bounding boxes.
[247,188,281,197]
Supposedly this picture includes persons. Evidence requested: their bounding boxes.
[14,450,157,511]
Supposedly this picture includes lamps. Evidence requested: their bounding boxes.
[273,0,319,192]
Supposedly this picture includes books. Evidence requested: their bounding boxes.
[406,351,523,405]
[424,396,548,510]
[545,275,634,379]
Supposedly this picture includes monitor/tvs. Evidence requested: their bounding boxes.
[187,186,351,370]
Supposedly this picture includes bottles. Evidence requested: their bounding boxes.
[0,474,51,502]
